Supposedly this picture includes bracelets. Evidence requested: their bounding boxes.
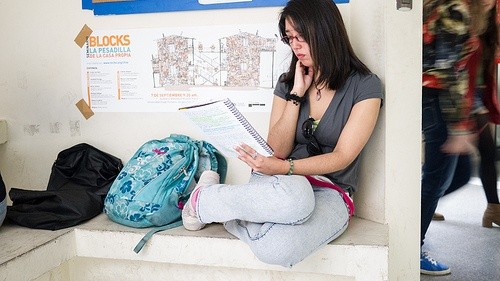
[285,92,307,104]
[285,159,294,176]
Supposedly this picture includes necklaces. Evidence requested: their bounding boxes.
[312,78,328,101]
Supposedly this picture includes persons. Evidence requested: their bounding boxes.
[420,0,500,276]
[181,0,382,269]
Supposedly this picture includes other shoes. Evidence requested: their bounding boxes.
[182,170,220,231]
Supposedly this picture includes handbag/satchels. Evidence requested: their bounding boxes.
[7,143,124,230]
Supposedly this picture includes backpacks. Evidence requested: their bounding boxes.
[103,134,227,228]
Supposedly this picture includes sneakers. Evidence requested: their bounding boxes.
[420,250,451,276]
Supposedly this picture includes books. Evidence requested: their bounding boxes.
[179,99,274,158]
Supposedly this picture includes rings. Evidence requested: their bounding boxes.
[252,152,258,158]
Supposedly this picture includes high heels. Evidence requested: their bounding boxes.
[482,203,500,227]
[432,212,444,220]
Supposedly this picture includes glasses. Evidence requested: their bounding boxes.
[280,33,306,45]
[302,118,322,156]
